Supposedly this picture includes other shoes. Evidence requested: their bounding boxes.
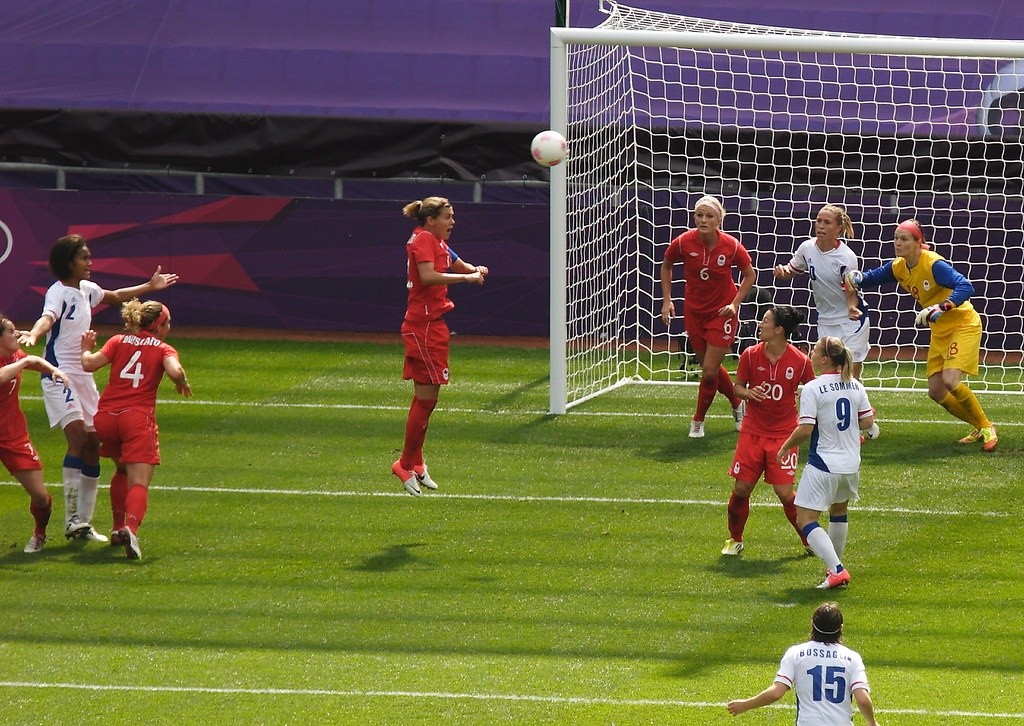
[118,524,141,561]
[110,529,121,547]
[65,522,91,539]
[75,522,109,544]
[25,527,47,555]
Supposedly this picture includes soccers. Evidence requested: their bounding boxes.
[531,130,567,167]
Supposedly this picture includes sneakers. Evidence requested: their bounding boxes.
[802,546,815,556]
[391,459,422,499]
[415,457,438,491]
[721,538,745,556]
[730,400,745,431]
[824,568,847,589]
[687,419,705,438]
[814,568,851,590]
[867,424,880,440]
[959,426,984,445]
[978,426,998,450]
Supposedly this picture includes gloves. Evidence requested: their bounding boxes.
[914,300,953,328]
[841,269,863,296]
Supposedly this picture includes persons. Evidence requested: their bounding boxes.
[0,313,69,553]
[391,196,489,497]
[777,337,873,589]
[721,305,876,556]
[17,233,179,544]
[80,297,192,561]
[728,602,879,726]
[845,219,998,450]
[773,204,873,444]
[661,195,757,438]
